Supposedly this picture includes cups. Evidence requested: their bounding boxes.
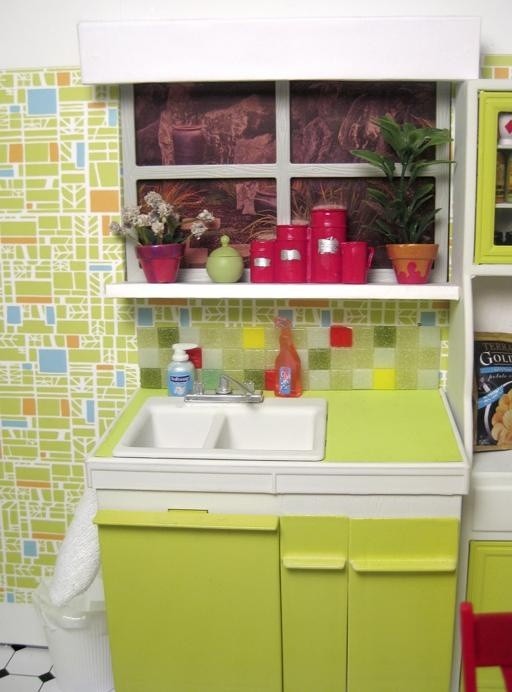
[250,205,374,285]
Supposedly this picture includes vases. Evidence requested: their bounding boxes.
[134,241,182,282]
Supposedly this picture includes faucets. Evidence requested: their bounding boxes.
[216,375,251,395]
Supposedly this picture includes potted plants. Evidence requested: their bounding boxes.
[351,116,457,284]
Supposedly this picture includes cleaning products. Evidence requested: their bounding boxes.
[274,317,302,396]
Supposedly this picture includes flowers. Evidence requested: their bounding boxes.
[108,191,216,247]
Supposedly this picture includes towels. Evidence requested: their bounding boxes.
[48,489,100,608]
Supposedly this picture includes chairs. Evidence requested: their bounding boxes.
[459,601,511,692]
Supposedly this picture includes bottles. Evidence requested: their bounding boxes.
[203,236,244,283]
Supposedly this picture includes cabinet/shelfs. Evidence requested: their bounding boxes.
[91,489,462,692]
[446,79,512,692]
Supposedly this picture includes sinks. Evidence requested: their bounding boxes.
[214,398,327,460]
[114,396,216,459]
